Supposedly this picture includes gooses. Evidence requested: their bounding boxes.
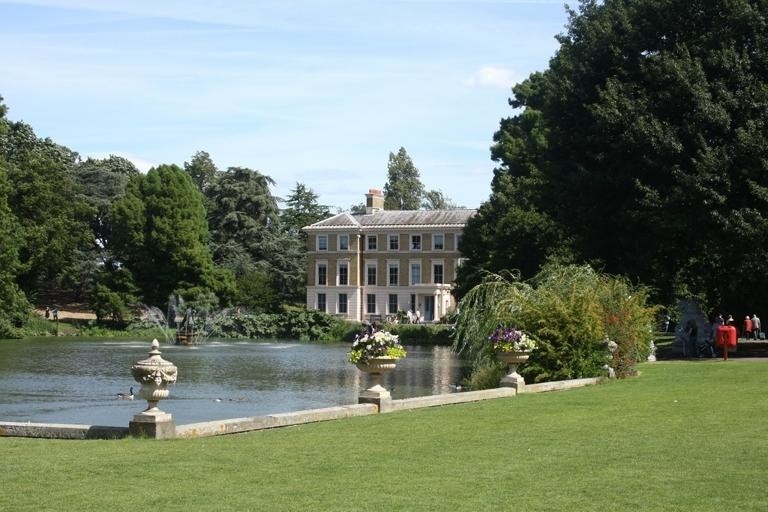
[114,387,134,401]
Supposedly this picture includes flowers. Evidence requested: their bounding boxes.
[488,327,538,355]
[347,323,408,364]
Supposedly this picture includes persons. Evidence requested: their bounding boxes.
[45,306,49,320]
[415,308,421,324]
[743,315,753,340]
[390,314,398,325]
[726,314,736,326]
[52,305,58,321]
[235,307,240,316]
[750,313,761,340]
[712,313,725,337]
[406,308,413,324]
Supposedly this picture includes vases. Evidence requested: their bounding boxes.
[494,351,529,378]
[356,355,398,371]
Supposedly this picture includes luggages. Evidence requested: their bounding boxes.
[759,329,765,340]
